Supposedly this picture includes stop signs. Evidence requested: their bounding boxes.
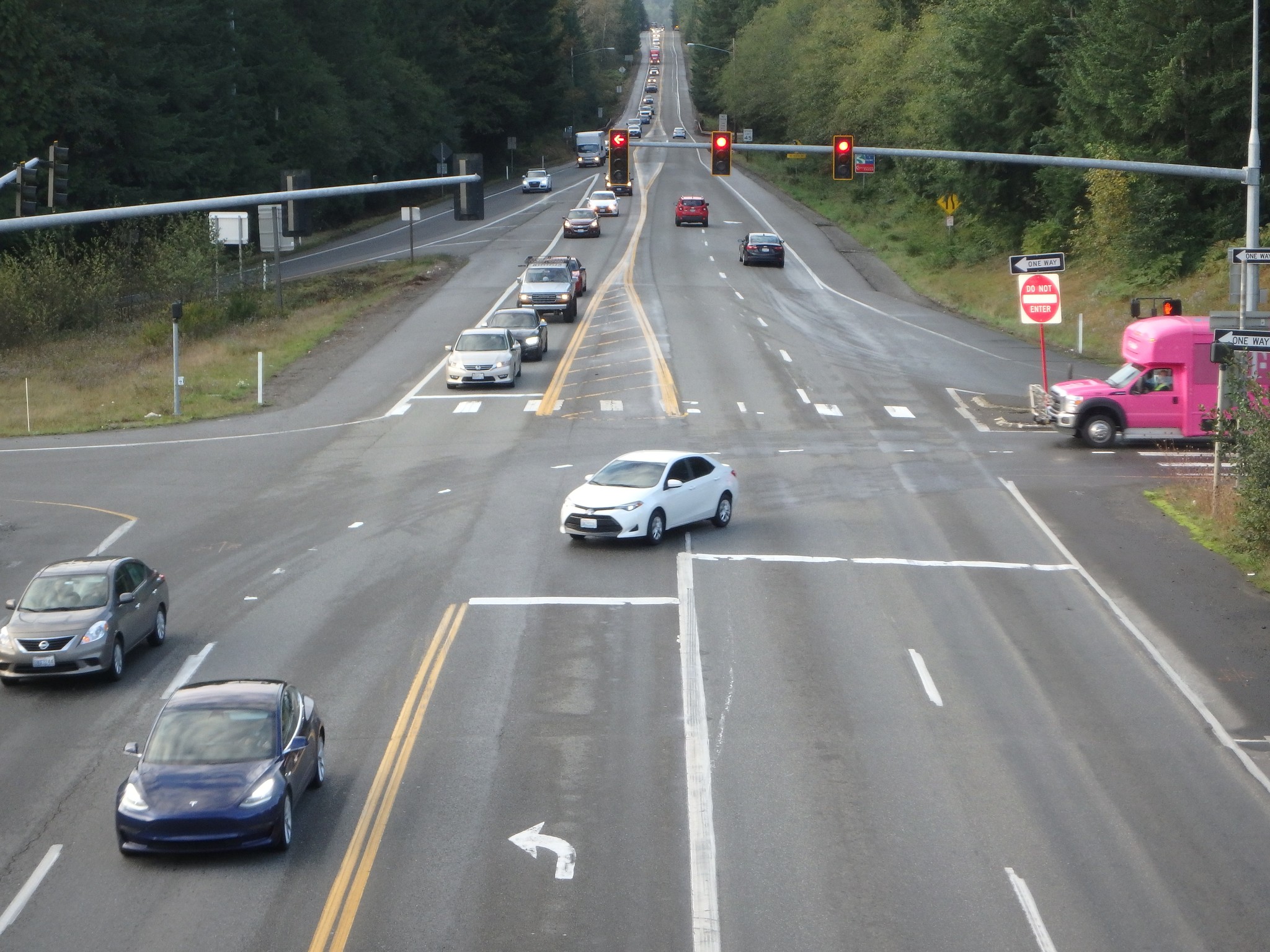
[1018,274,1062,325]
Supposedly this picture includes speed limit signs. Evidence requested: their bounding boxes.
[744,128,753,142]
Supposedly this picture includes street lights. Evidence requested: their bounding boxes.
[570,46,615,130]
[687,38,738,143]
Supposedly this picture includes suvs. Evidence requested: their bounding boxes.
[516,262,580,323]
[674,196,711,227]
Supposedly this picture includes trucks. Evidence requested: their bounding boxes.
[574,131,606,168]
[1029,315,1270,448]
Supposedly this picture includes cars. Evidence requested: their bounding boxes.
[542,256,588,298]
[522,169,553,193]
[737,233,785,270]
[673,127,686,139]
[0,553,171,684]
[113,679,328,858]
[587,191,621,218]
[481,308,550,360]
[561,450,739,547]
[560,207,602,239]
[443,328,524,388]
[603,20,680,157]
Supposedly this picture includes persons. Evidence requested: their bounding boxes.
[521,314,534,327]
[1144,370,1171,393]
[48,580,76,608]
[585,213,592,218]
[92,578,120,603]
[491,336,506,349]
[552,269,568,283]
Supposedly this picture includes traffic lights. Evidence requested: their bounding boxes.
[610,129,629,186]
[1163,299,1183,317]
[833,135,854,180]
[712,131,731,177]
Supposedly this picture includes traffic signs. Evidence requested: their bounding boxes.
[1009,253,1065,273]
[1233,249,1270,264]
[1214,329,1270,353]
